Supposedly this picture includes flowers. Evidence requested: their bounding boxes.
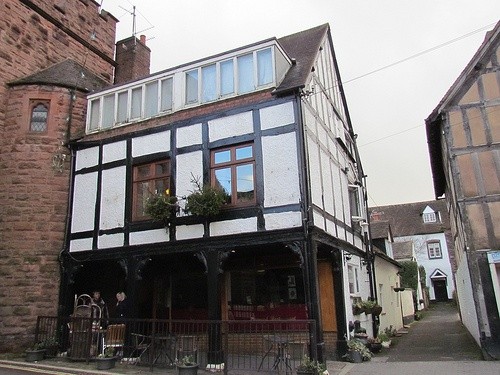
[175,355,197,366]
[298,358,325,372]
[99,348,117,358]
[356,299,377,308]
[347,338,374,360]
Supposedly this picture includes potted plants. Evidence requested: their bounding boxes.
[25,336,59,361]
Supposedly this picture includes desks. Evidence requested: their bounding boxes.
[70,329,108,355]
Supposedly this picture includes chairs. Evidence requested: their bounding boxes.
[128,331,175,366]
[256,334,293,375]
[101,323,127,356]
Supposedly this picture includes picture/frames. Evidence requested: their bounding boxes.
[289,288,297,299]
[288,274,295,287]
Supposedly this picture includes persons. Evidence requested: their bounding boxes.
[87,290,130,357]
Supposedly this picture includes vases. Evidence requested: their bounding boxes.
[357,306,365,313]
[96,356,115,370]
[177,365,199,375]
[349,349,363,362]
[365,309,374,314]
[296,371,323,375]
